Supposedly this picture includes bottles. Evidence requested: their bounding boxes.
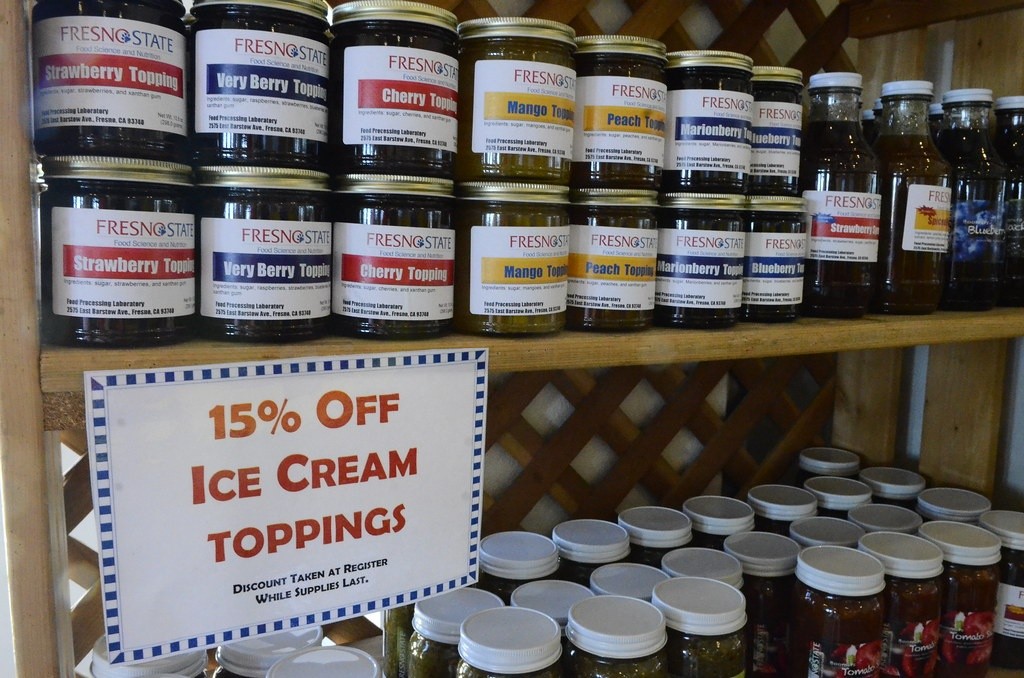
[866,81,1023,315]
[799,72,881,320]
